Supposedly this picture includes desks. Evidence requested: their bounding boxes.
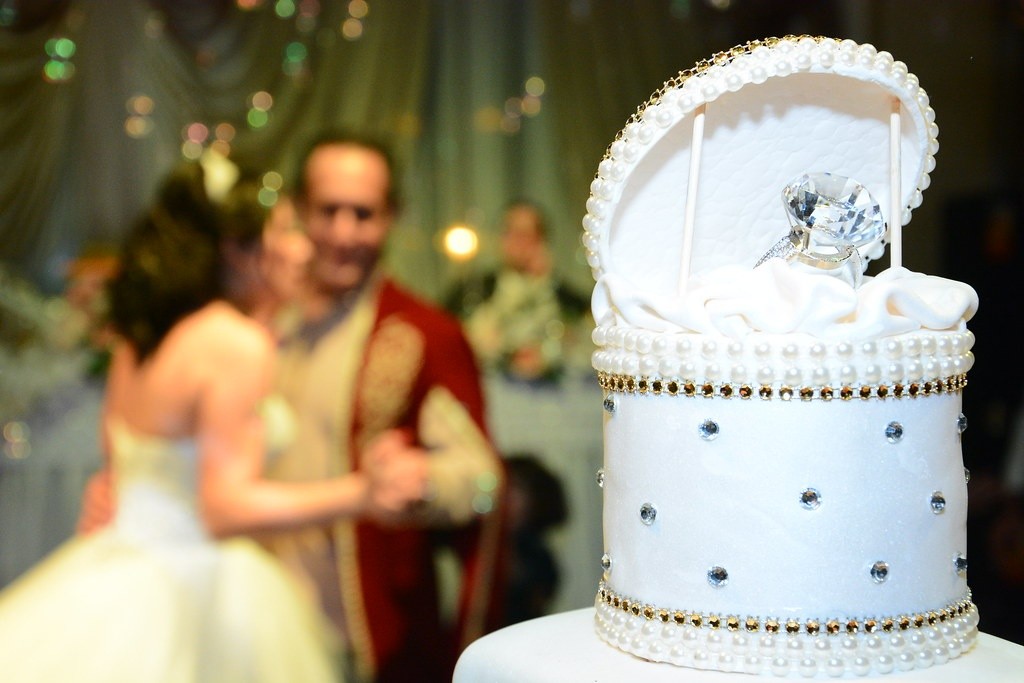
[451,604,1024,683]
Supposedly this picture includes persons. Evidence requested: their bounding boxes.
[29,147,427,683]
[76,127,507,683]
[917,194,1024,571]
[439,198,597,616]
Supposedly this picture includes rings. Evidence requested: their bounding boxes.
[747,169,891,291]
